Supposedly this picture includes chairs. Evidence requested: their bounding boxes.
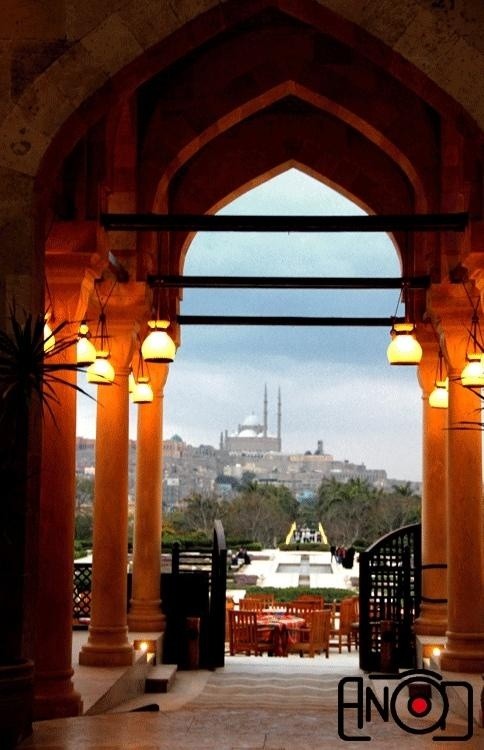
[223,593,391,658]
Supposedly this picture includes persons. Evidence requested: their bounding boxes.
[293,521,322,544]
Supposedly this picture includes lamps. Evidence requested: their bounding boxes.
[39,266,176,403]
[386,264,484,408]
